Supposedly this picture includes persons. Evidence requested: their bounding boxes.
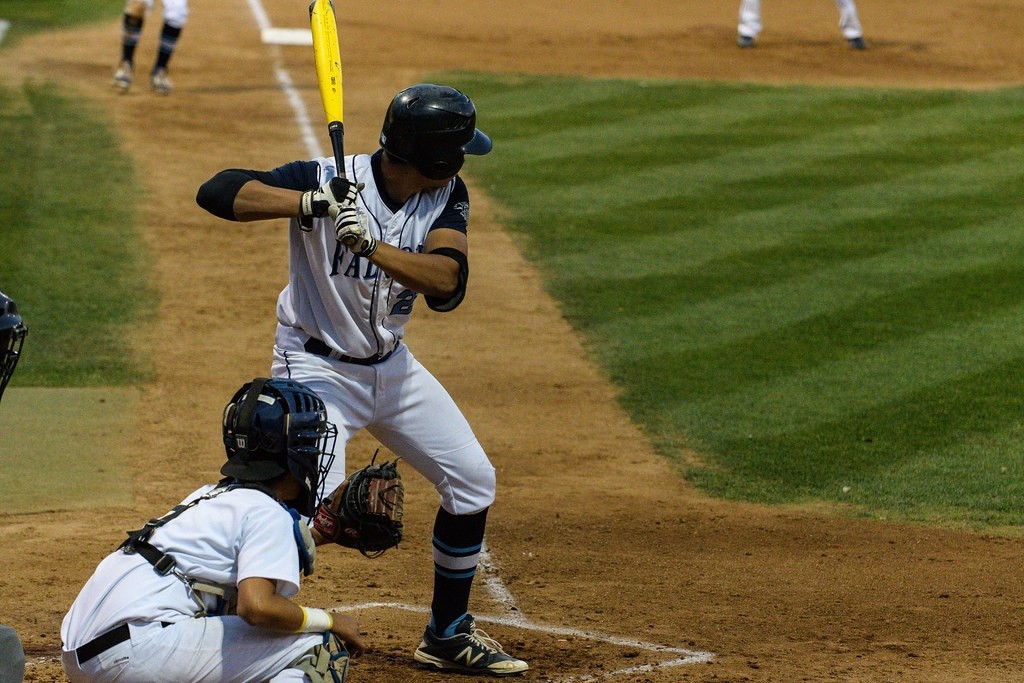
[0,284,29,682]
[113,0,190,95]
[737,0,867,50]
[195,84,531,677]
[59,377,404,683]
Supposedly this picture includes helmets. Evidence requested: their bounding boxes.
[379,83,493,180]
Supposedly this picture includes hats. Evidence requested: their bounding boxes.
[221,377,325,481]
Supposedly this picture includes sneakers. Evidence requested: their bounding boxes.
[114,60,132,87]
[414,614,528,674]
[151,72,171,94]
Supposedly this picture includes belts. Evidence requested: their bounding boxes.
[304,336,400,365]
[76,620,171,667]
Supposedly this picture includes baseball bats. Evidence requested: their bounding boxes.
[306,0,359,248]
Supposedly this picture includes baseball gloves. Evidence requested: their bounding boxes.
[313,446,406,559]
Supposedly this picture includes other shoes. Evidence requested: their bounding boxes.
[737,34,754,48]
[848,37,865,52]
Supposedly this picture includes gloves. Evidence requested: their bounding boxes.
[328,204,378,260]
[298,177,365,231]
